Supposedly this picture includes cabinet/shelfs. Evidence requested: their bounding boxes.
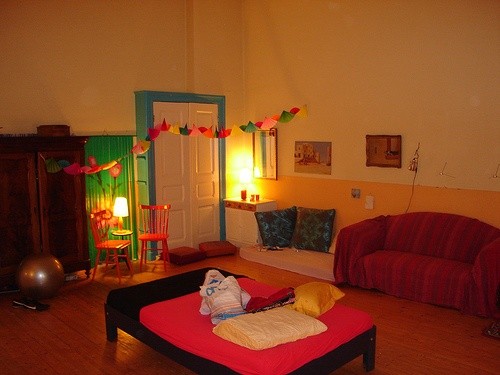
[223,197,275,246]
[0,135,92,286]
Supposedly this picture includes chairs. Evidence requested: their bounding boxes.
[89,210,133,284]
[138,204,171,273]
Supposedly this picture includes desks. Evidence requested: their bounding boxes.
[105,267,376,375]
[111,229,132,270]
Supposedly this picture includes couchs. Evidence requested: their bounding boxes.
[334,212,500,319]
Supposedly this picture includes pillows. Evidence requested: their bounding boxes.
[290,208,335,252]
[287,281,346,318]
[254,207,297,251]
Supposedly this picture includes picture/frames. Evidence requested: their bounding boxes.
[365,134,401,168]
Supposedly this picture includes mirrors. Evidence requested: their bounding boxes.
[252,129,277,181]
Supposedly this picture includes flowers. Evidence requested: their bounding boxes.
[63,156,147,238]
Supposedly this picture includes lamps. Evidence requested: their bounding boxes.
[408,151,420,171]
[113,197,128,233]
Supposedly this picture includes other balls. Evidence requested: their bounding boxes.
[18,252,65,300]
